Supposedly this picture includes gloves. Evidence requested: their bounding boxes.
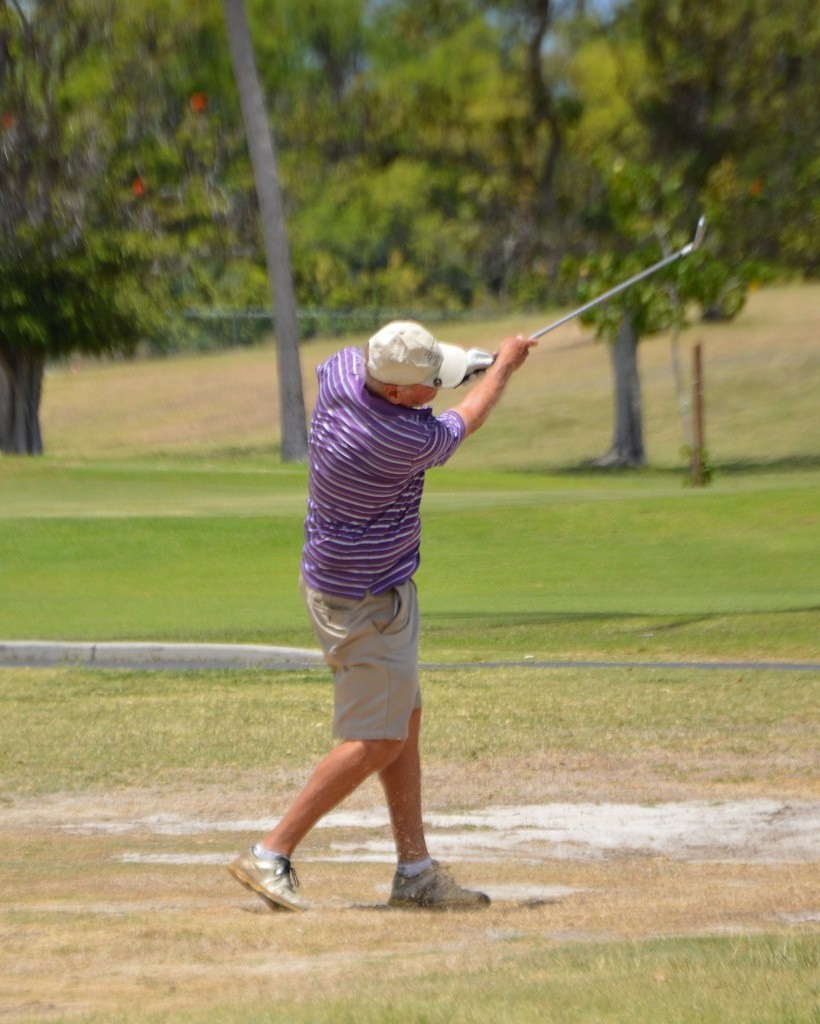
[463,347,494,378]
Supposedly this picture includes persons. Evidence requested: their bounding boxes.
[224,320,540,915]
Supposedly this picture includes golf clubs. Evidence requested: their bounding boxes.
[523,215,707,341]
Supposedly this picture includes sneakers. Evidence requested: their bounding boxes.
[390,860,491,909]
[227,844,307,913]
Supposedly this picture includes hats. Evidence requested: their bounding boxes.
[366,319,469,390]
